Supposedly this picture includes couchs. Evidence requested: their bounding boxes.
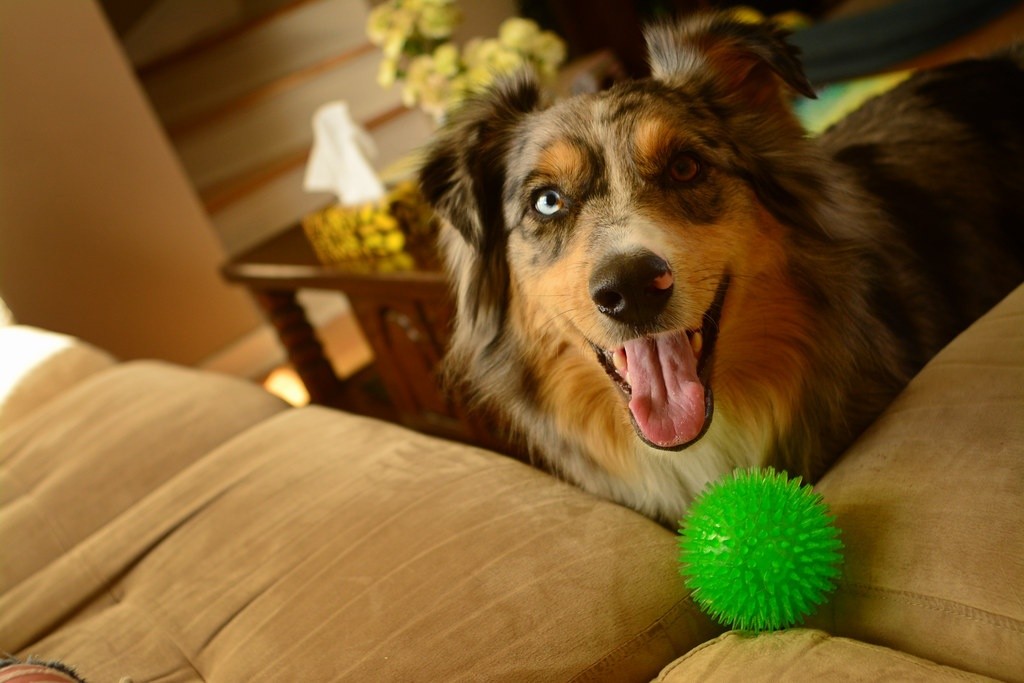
[0,282,1024,682]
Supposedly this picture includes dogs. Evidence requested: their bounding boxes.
[413,7,1023,538]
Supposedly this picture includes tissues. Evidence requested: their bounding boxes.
[301,99,444,260]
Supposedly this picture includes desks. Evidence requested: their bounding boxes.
[225,202,491,441]
